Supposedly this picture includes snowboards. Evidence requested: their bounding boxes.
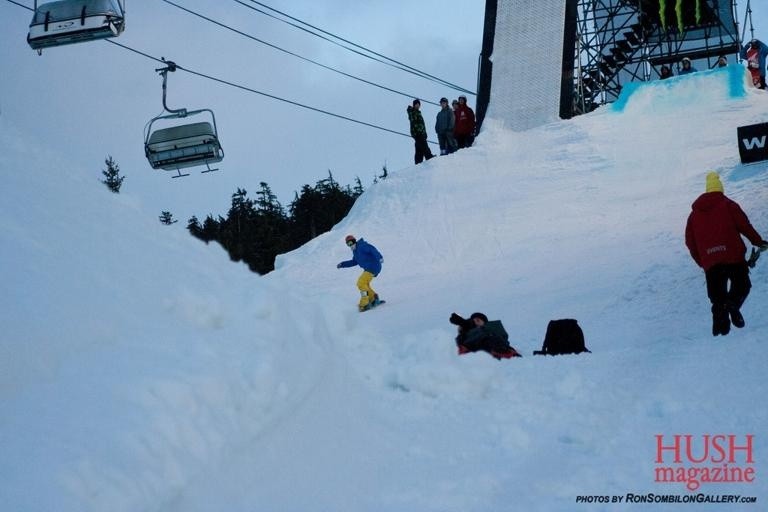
[358,300,386,313]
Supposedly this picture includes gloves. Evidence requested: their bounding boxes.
[336,264,342,268]
[378,259,383,263]
[757,240,767,251]
[746,247,759,268]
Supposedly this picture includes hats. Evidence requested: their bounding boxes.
[705,172,722,193]
[345,235,353,242]
[717,56,726,64]
[439,95,466,105]
[412,100,420,107]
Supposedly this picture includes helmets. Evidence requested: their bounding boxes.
[681,56,690,63]
[660,66,667,71]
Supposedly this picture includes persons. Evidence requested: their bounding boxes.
[456,313,522,361]
[454,97,474,148]
[718,56,727,67]
[685,172,768,336]
[407,99,435,164]
[337,235,384,310]
[452,100,459,110]
[435,98,457,155]
[660,66,674,79]
[678,57,698,75]
[740,39,768,89]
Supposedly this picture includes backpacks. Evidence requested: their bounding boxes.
[532,319,592,355]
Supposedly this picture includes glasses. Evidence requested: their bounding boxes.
[346,240,353,246]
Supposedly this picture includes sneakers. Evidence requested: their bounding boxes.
[729,311,744,327]
